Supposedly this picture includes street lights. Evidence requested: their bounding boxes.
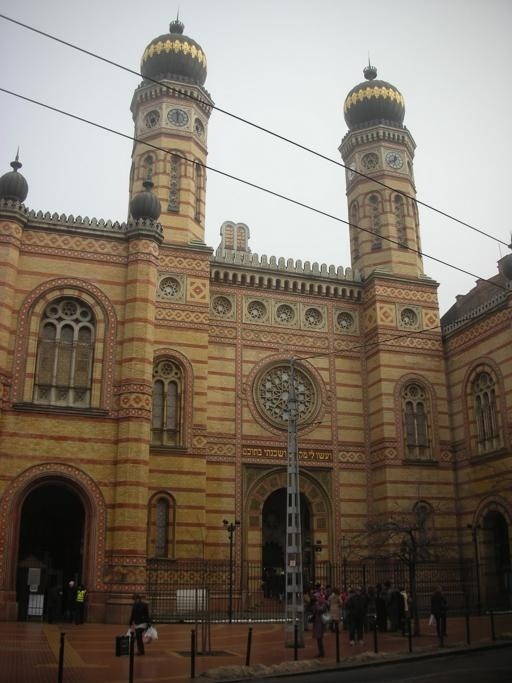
[306,537,322,591]
[468,522,482,618]
[223,517,241,624]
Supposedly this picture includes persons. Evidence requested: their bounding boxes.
[128,591,151,655]
[431,584,449,646]
[73,584,88,625]
[302,582,413,658]
[64,581,75,621]
